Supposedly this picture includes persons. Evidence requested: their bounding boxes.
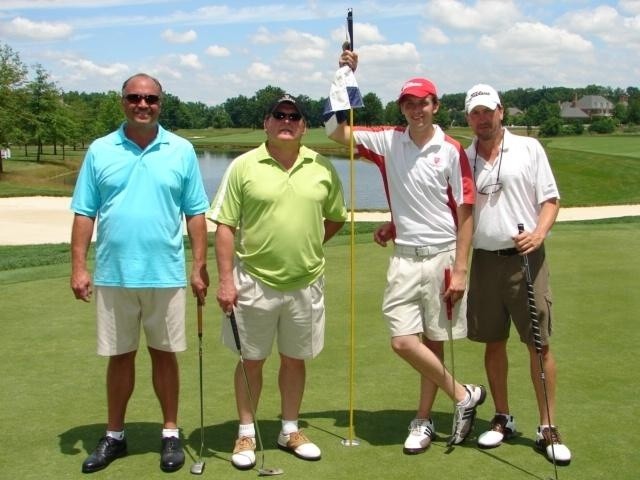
[374,83,572,465]
[68,72,213,473]
[204,93,350,470]
[326,49,487,454]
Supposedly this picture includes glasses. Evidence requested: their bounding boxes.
[123,94,161,105]
[270,110,303,123]
[473,180,504,198]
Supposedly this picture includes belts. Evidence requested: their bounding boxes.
[393,239,460,259]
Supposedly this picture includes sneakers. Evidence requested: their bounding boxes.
[80,434,129,474]
[159,435,187,472]
[402,416,436,455]
[476,413,516,448]
[230,435,260,471]
[534,424,572,466]
[450,382,487,446]
[276,428,322,461]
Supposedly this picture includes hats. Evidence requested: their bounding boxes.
[397,77,452,111]
[463,83,503,116]
[266,93,306,119]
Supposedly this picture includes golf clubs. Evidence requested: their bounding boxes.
[189,290,206,476]
[226,311,283,476]
[517,223,561,479]
[442,255,456,453]
